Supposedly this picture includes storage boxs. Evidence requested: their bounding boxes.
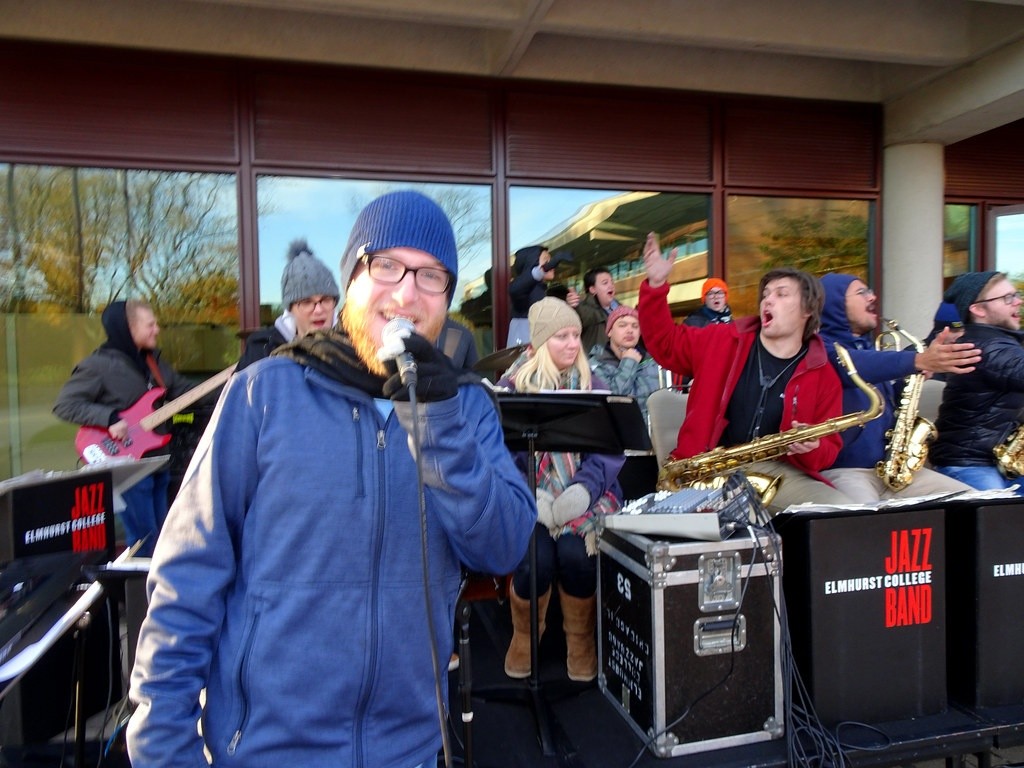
[594,511,784,760]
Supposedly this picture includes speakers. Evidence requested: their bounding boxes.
[0,469,124,748]
[773,503,1024,731]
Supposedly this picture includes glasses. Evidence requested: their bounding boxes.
[970,288,1023,305]
[706,289,726,299]
[844,287,873,301]
[290,295,338,315]
[360,252,452,296]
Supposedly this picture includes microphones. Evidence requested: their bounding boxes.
[382,317,417,388]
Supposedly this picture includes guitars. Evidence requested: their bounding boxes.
[73,360,239,468]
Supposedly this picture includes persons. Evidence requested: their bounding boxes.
[922,269,1024,502]
[230,235,340,380]
[574,267,640,361]
[888,304,962,412]
[126,187,534,768]
[583,304,661,526]
[501,245,581,356]
[433,300,489,673]
[638,232,850,510]
[492,293,633,681]
[810,272,982,475]
[677,277,736,396]
[50,298,201,582]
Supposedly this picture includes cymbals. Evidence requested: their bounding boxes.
[473,343,530,371]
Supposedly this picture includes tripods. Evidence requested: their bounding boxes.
[466,389,654,764]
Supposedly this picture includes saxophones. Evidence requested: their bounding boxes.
[657,340,887,510]
[874,315,939,493]
[991,424,1024,481]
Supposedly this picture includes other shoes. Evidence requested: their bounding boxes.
[448,653,460,671]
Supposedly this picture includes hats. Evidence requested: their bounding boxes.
[933,301,965,330]
[604,302,638,335]
[942,271,1000,324]
[701,278,728,306]
[281,239,339,310]
[338,191,457,310]
[528,295,582,351]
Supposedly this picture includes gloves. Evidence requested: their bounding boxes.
[543,249,573,274]
[383,334,458,403]
[553,482,591,528]
[536,490,554,528]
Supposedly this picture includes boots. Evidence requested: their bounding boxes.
[504,575,551,678]
[559,585,599,682]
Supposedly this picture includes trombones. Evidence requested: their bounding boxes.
[875,330,901,355]
